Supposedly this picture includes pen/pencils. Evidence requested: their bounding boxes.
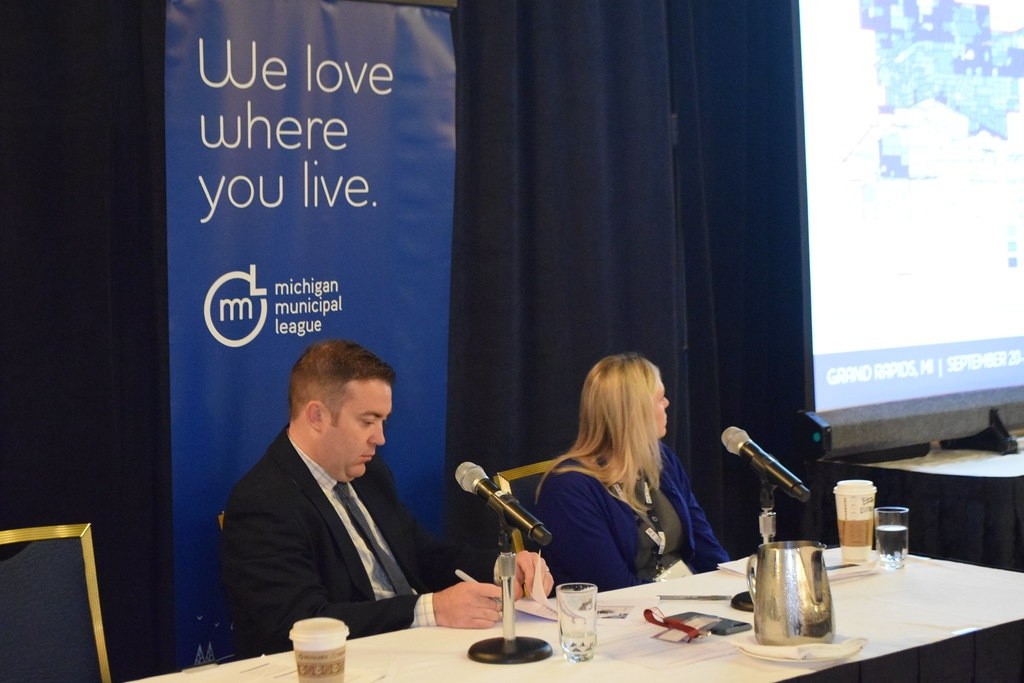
[454,568,505,606]
[657,593,730,601]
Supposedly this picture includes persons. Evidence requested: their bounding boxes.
[534,353,729,593]
[218,341,554,658]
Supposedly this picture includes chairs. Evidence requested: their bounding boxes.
[0,523,112,683]
[493,460,553,597]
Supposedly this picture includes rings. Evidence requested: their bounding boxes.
[545,569,549,572]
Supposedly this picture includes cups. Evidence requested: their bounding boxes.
[556,582,598,663]
[289,617,350,683]
[833,480,878,565]
[874,507,909,571]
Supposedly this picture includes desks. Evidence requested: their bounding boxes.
[130,546,1024,683]
[803,437,1024,683]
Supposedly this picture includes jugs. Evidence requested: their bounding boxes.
[746,540,835,646]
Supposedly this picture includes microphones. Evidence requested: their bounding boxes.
[456,461,551,545]
[721,427,809,501]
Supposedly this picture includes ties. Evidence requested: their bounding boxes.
[333,481,413,595]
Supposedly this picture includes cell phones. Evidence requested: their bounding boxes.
[664,611,752,635]
